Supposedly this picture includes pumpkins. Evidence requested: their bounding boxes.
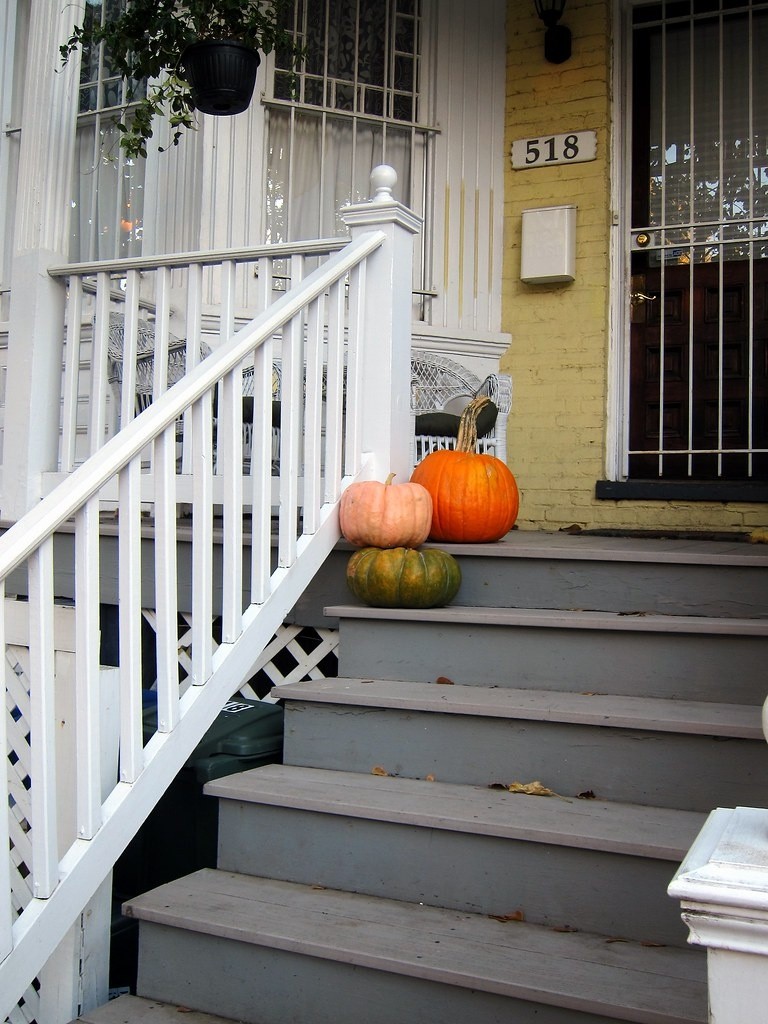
[410,395,519,544]
[338,472,433,549]
[346,546,461,609]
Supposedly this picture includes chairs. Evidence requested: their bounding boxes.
[96,312,282,479]
[297,350,514,465]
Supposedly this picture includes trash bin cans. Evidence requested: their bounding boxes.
[106,698,284,1003]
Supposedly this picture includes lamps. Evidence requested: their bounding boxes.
[532,0,572,64]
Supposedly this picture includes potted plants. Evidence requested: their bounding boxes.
[52,0,316,176]
